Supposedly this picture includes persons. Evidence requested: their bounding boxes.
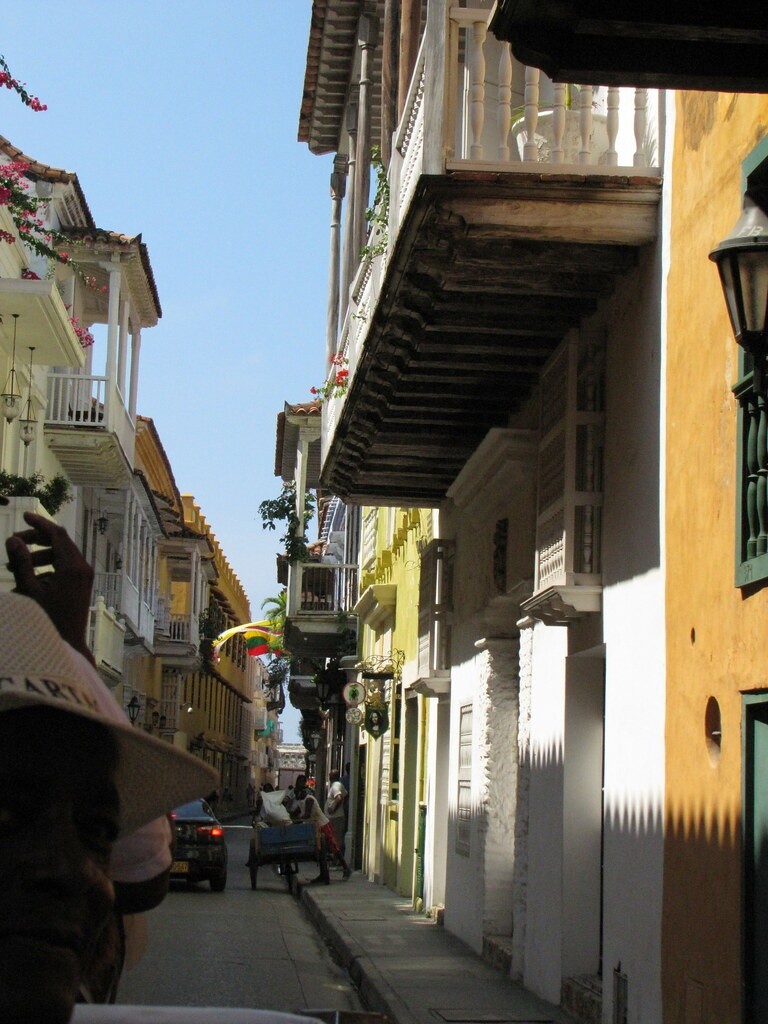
[1,512,220,1024]
[323,768,348,868]
[289,784,352,883]
[283,774,314,823]
[370,712,383,733]
[251,783,275,825]
[223,786,232,814]
[246,783,255,807]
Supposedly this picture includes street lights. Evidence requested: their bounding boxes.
[126,694,142,725]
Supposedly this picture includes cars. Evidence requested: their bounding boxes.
[169,797,227,890]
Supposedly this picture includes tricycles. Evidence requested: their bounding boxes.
[245,808,326,891]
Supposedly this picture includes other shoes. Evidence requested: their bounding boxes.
[310,875,330,885]
[342,869,353,881]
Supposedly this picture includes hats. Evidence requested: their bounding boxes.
[0,590,221,842]
